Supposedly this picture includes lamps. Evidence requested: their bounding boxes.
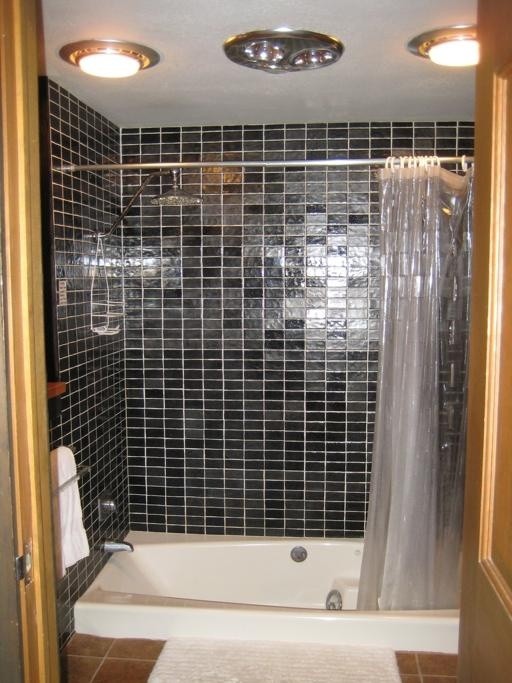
[57,38,162,81]
[406,24,479,66]
[218,28,343,75]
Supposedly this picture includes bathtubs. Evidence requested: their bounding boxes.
[73,530,462,656]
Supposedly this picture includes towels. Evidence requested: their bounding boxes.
[47,448,92,575]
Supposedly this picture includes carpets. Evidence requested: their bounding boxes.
[145,647,401,683]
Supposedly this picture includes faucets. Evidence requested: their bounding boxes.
[104,538,134,555]
